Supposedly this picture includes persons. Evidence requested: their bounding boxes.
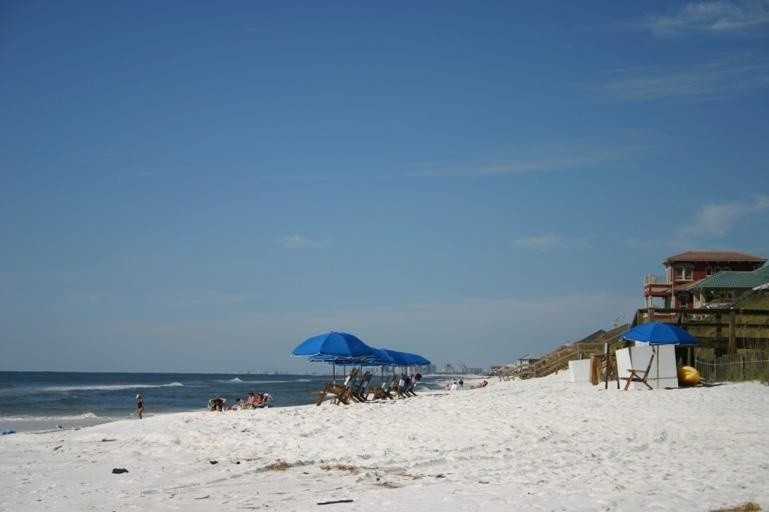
[136,392,144,419]
[458,378,464,392]
[450,378,457,392]
[207,390,271,411]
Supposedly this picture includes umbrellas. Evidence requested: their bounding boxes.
[621,318,699,389]
[290,328,431,383]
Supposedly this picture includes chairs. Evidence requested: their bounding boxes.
[311,367,418,406]
[620,354,654,391]
